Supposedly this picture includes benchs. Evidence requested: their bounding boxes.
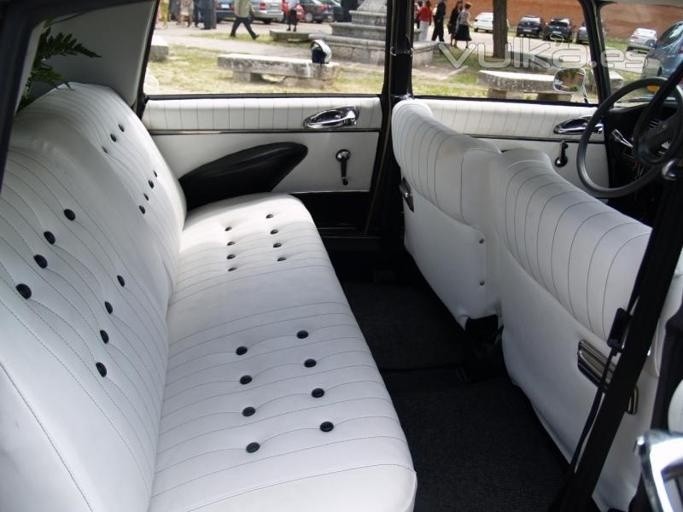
[217,53,341,88]
[269,30,313,42]
[478,69,573,102]
[0,82,419,512]
[589,69,624,93]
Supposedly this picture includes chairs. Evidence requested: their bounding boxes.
[490,148,683,512]
[390,98,501,363]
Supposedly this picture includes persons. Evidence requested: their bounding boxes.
[231,0,261,41]
[285,0,300,32]
[281,0,290,24]
[161,0,219,29]
[414,0,472,48]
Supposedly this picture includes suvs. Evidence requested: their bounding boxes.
[516,14,605,44]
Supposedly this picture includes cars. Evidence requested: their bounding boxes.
[626,28,658,51]
[474,11,510,34]
[194,0,343,24]
[640,20,683,97]
